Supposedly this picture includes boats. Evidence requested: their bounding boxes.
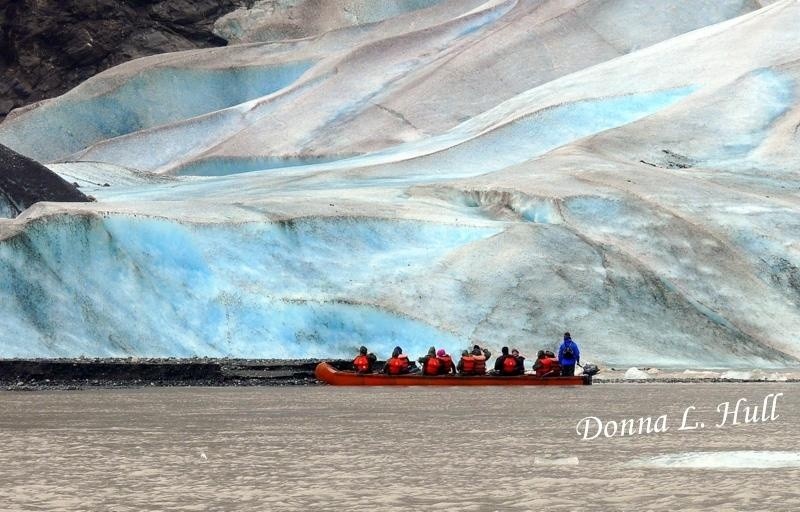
[315,361,601,387]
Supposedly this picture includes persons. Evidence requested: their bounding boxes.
[557,331,581,377]
[347,344,562,377]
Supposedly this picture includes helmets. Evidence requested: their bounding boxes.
[437,349,445,356]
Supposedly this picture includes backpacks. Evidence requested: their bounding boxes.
[563,342,573,358]
